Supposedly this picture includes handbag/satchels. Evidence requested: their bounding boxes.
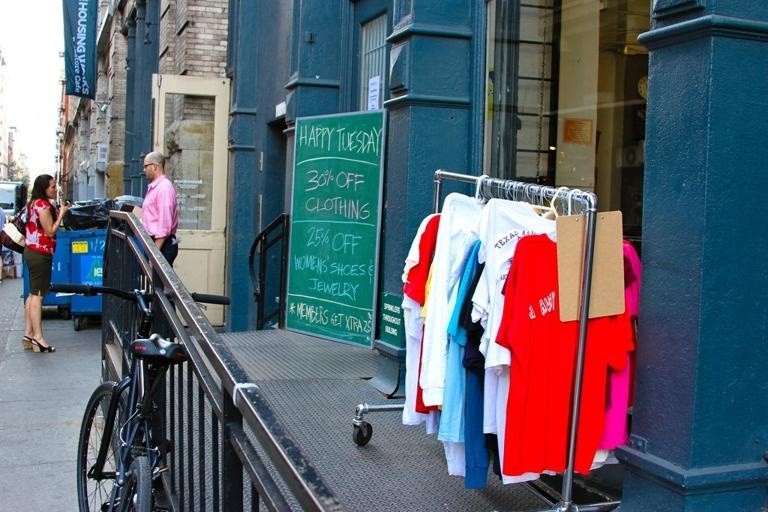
[0,216,27,254]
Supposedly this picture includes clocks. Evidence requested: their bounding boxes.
[637,75,647,99]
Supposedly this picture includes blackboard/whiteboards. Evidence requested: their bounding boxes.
[284,108,387,350]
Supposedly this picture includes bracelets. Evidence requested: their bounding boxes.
[55,223,60,227]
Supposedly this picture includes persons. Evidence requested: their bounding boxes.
[22,173,71,351]
[142,152,180,342]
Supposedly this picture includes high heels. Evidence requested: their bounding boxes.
[22,335,55,353]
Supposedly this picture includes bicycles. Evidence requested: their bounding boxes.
[49,283,231,512]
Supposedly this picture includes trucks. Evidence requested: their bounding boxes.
[0,180,27,216]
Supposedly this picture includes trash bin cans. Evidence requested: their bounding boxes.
[20,195,146,330]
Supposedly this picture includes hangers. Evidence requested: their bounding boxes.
[476,174,597,220]
